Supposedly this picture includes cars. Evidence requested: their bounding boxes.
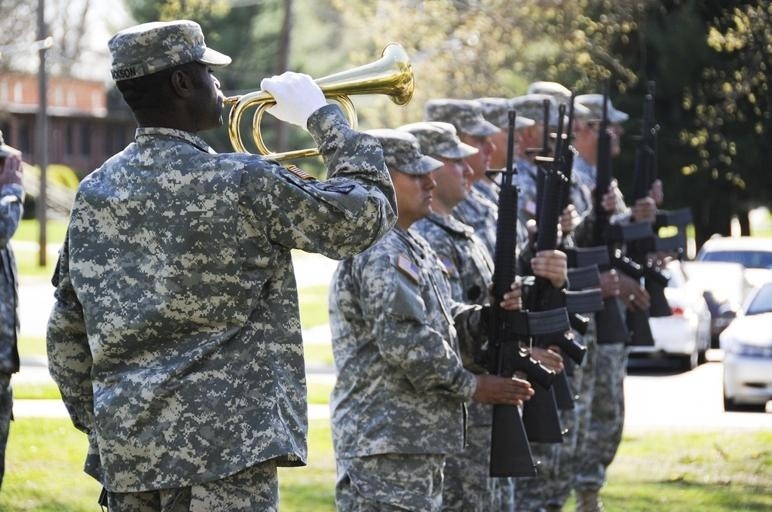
[681,259,744,311]
[623,266,710,370]
[718,279,772,411]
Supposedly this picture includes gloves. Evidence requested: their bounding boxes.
[260,70,328,132]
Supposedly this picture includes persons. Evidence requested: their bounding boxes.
[327,82,664,511]
[1,130,25,490]
[46,20,399,511]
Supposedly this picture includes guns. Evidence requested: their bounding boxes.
[523,103,606,443]
[534,101,577,411]
[616,94,658,346]
[561,95,609,334]
[487,112,571,477]
[591,92,634,345]
[637,82,670,314]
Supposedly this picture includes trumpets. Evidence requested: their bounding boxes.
[223,42,415,161]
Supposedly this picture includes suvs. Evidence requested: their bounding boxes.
[702,234,772,283]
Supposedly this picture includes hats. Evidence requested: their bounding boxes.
[106,20,231,81]
[361,82,629,175]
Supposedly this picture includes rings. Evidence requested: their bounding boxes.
[628,294,635,301]
